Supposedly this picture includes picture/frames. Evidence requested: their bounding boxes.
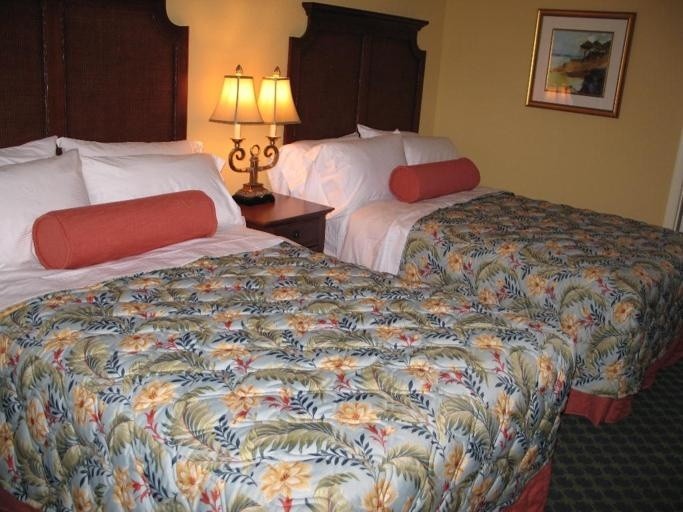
[523,7,639,120]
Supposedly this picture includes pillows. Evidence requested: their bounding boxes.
[356,124,418,140]
[280,130,409,218]
[266,131,360,197]
[401,134,461,167]
[387,156,480,204]
[0,134,56,168]
[56,134,202,162]
[31,191,222,270]
[78,152,249,229]
[0,148,92,275]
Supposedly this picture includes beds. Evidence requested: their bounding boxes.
[1,0,577,512]
[266,2,681,425]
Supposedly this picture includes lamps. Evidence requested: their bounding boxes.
[207,64,301,203]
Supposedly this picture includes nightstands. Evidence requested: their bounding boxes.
[234,190,335,253]
